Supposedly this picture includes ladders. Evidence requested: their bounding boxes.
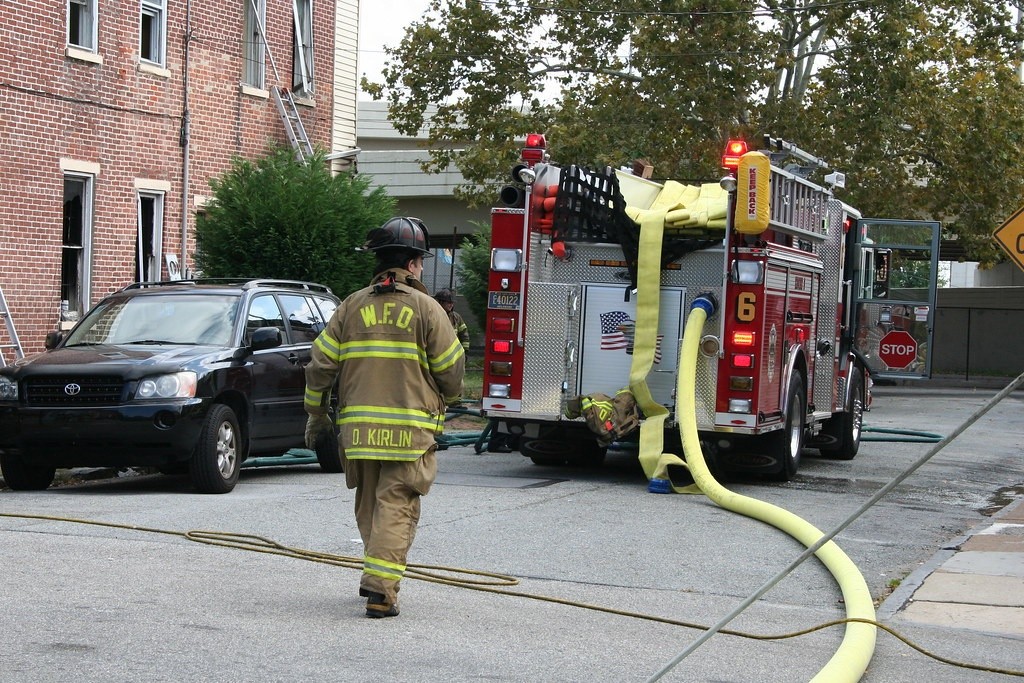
[0,286,25,369]
[272,85,315,167]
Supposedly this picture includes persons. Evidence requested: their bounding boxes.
[304,215,465,618]
[434,289,470,353]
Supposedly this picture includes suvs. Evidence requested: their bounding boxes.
[1,279,356,497]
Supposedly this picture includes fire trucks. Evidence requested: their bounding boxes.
[484,130,940,472]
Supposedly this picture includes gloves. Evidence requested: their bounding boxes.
[304,412,333,449]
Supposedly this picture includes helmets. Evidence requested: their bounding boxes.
[360,216,434,258]
[434,288,457,303]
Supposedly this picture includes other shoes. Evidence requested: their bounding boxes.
[366,591,400,618]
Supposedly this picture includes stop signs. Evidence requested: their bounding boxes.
[878,330,917,369]
